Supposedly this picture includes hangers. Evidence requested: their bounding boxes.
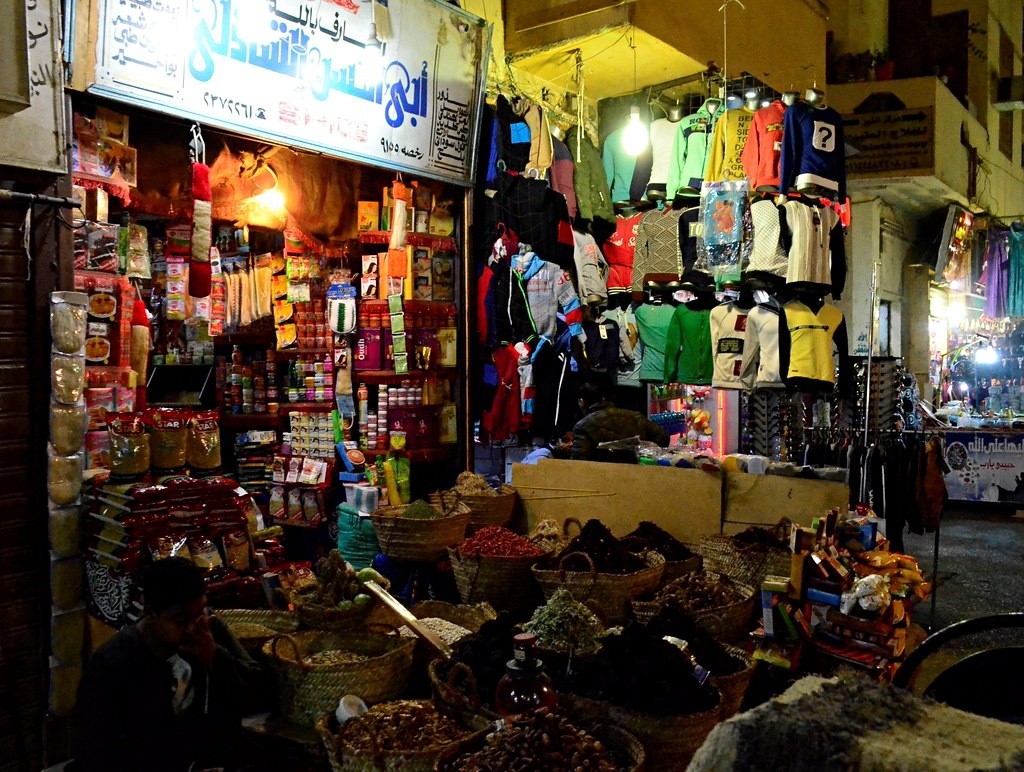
[806,427,942,462]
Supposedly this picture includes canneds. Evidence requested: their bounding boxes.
[357,312,391,328]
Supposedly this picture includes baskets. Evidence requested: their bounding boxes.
[434,715,645,772]
[532,535,791,643]
[522,623,757,772]
[370,500,471,560]
[316,701,491,772]
[273,571,390,629]
[446,536,555,609]
[383,600,497,674]
[214,610,298,653]
[429,483,516,532]
[263,629,416,726]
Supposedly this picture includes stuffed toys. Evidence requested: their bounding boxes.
[682,385,712,405]
[677,408,713,458]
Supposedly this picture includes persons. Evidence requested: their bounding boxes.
[76,557,331,772]
[886,412,924,537]
[933,414,951,427]
[506,425,573,487]
[479,79,854,435]
[570,382,670,464]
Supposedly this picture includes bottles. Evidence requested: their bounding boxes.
[364,455,386,486]
[231,343,243,363]
[495,632,556,717]
[357,382,368,400]
[358,400,368,425]
[287,354,320,387]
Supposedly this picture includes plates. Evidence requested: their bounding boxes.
[88,293,117,319]
[85,337,111,361]
